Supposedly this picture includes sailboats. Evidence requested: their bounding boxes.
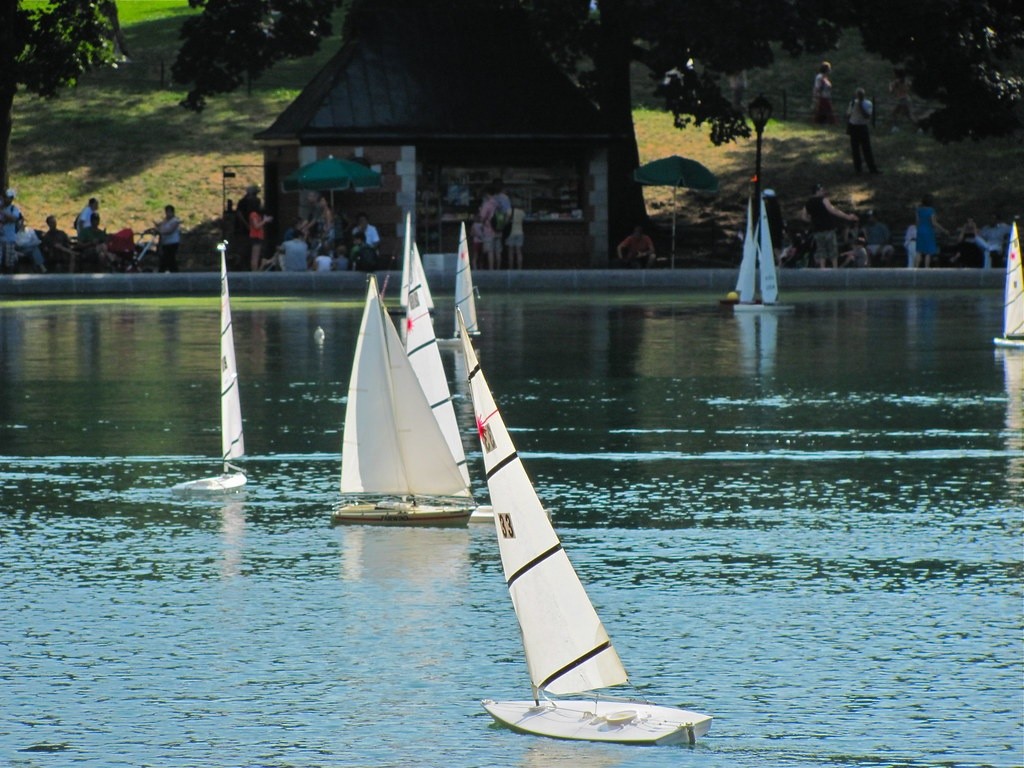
[329,268,479,524]
[992,221,1024,350]
[169,240,249,494]
[455,304,714,749]
[394,209,555,526]
[433,219,482,351]
[733,187,796,312]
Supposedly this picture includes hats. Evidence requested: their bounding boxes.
[762,189,775,198]
[91,213,99,220]
[855,237,866,245]
[6,188,15,197]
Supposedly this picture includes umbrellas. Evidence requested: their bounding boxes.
[283,155,384,273]
[625,156,719,269]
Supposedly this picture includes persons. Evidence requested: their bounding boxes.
[845,87,879,174]
[0,179,1016,275]
[812,61,837,126]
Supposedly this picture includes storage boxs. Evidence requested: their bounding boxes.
[423,253,456,271]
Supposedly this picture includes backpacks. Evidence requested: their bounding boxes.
[488,202,506,233]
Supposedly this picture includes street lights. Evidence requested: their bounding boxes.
[748,94,772,229]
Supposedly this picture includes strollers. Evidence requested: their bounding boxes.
[106,226,159,272]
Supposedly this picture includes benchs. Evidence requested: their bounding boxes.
[890,231,957,265]
